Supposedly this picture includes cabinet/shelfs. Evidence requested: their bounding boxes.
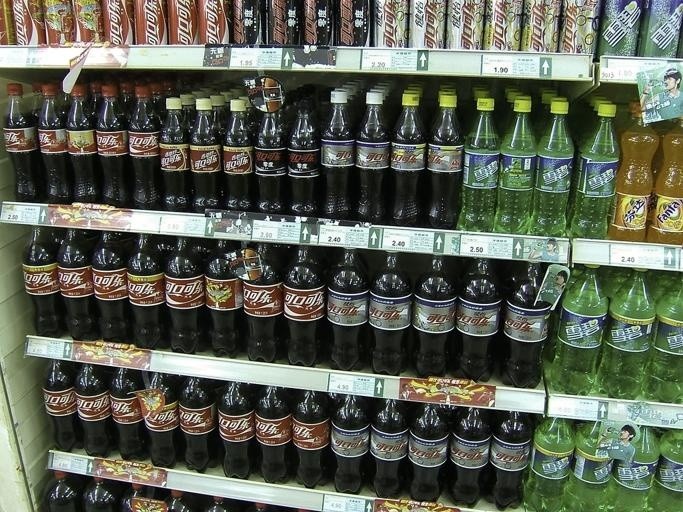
[0,43,683,512]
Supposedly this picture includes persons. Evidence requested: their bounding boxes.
[596,425,637,464]
[527,239,559,262]
[538,270,567,305]
[639,68,683,121]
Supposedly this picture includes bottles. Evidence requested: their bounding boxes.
[20,225,681,409]
[41,354,683,512]
[41,464,286,512]
[2,79,683,247]
